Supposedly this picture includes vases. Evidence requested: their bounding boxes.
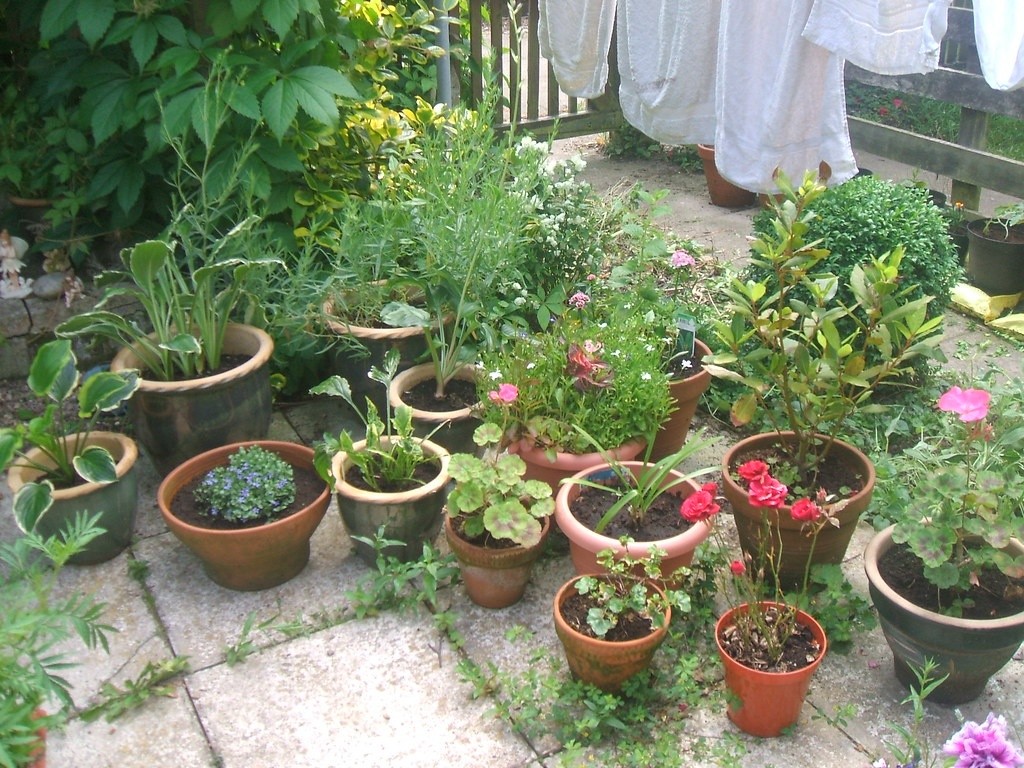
[714,601,827,738]
[507,433,652,519]
[637,327,713,465]
[697,144,872,209]
[158,440,333,590]
[943,216,971,265]
[443,499,549,609]
[864,516,1024,703]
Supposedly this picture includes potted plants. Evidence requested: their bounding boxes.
[0,1,559,569]
[904,165,946,209]
[701,161,949,591]
[966,204,1024,295]
[552,408,714,693]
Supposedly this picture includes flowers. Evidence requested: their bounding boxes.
[192,443,296,522]
[679,461,840,661]
[940,201,967,229]
[935,712,1024,768]
[443,188,701,545]
[880,387,1024,618]
